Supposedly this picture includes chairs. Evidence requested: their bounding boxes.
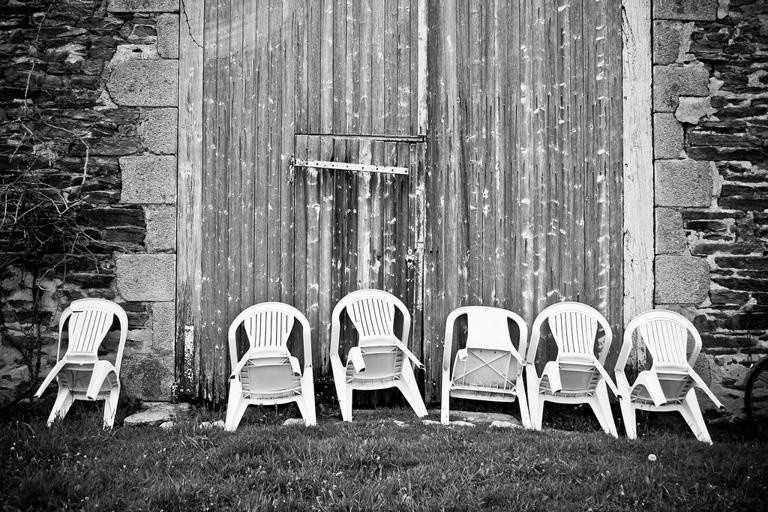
[614,309,726,446]
[33,297,128,431]
[329,289,428,423]
[525,301,622,439]
[440,305,532,428]
[223,301,317,433]
